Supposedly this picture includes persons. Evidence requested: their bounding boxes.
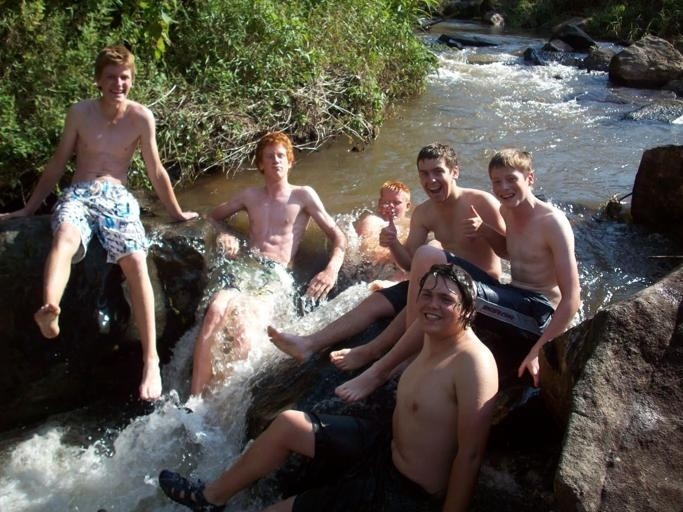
[267,143,509,370]
[159,263,500,512]
[0,43,198,402]
[327,179,412,301]
[183,131,348,414]
[334,147,581,403]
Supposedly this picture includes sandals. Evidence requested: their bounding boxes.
[158,470,226,512]
